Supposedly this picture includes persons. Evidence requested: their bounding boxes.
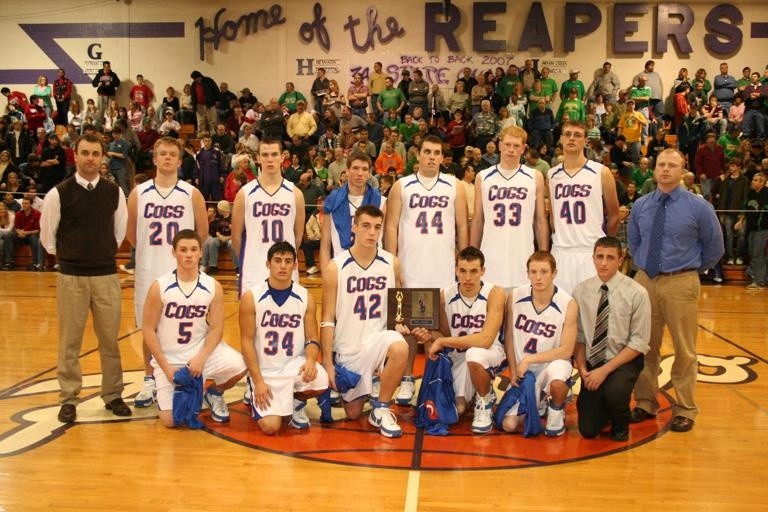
[1,57,768,286]
[40,122,725,441]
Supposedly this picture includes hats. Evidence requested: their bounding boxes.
[626,99,635,104]
[401,69,410,75]
[240,88,250,93]
[569,69,581,74]
[166,109,175,115]
[465,145,474,152]
[296,99,305,105]
[142,118,152,124]
[217,200,231,212]
[49,133,58,139]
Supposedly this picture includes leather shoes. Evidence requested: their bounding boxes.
[671,415,694,432]
[629,407,657,423]
[609,422,629,442]
[58,404,77,423]
[105,396,133,416]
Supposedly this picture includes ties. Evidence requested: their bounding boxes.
[645,193,669,279]
[86,182,94,193]
[587,284,611,367]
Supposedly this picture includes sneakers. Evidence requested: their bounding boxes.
[726,259,734,266]
[287,397,311,430]
[736,257,744,266]
[396,375,416,402]
[328,388,342,405]
[538,391,548,417]
[367,406,402,438]
[471,391,495,433]
[34,263,44,270]
[118,264,135,275]
[242,377,253,405]
[202,387,230,423]
[53,262,60,270]
[544,401,568,437]
[134,375,157,408]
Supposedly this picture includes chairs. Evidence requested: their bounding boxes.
[665,135,677,149]
[180,123,195,135]
[190,138,201,152]
[640,136,652,155]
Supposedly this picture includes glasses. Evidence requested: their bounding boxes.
[561,131,586,138]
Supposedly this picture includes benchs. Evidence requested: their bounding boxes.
[0,245,57,269]
[297,249,329,272]
[115,239,133,270]
[219,249,235,271]
